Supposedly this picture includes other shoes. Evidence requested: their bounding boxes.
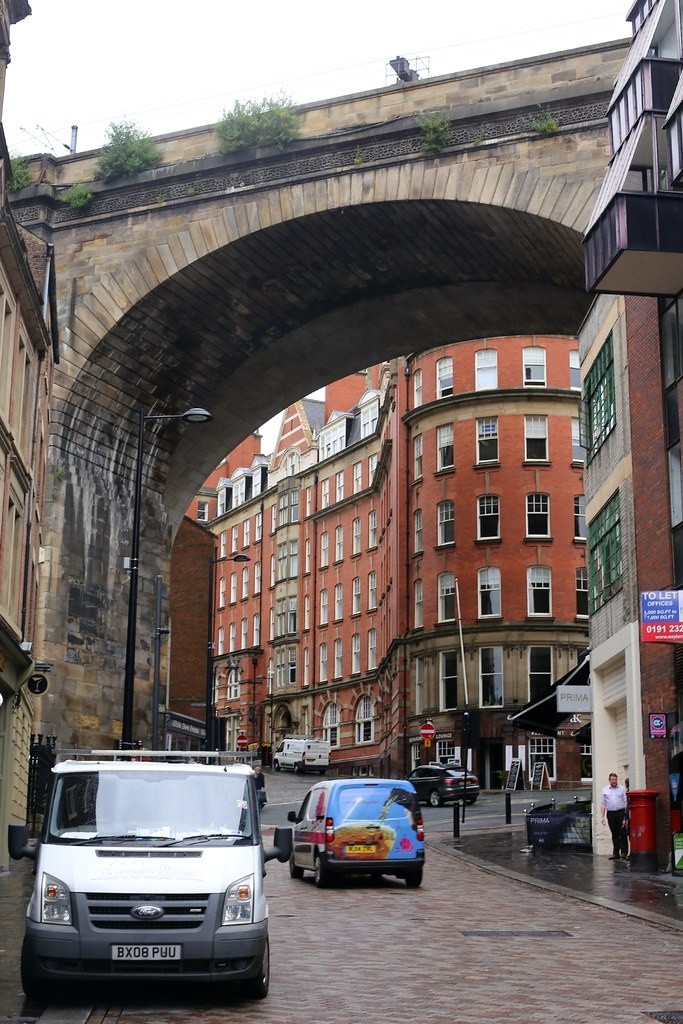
[260,803,266,809]
[609,855,620,859]
[621,853,630,860]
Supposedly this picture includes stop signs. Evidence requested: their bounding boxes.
[237,736,248,747]
[419,724,436,740]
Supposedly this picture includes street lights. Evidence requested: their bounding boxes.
[120,405,212,762]
[204,554,250,753]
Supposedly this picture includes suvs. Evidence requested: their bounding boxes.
[402,764,479,807]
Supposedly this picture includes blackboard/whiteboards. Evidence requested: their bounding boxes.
[531,762,551,788]
[505,758,525,790]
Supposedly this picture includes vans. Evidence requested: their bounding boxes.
[287,778,424,890]
[8,747,293,1001]
[275,734,330,775]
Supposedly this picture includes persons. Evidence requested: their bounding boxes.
[622,777,631,861]
[600,773,627,861]
[253,767,265,812]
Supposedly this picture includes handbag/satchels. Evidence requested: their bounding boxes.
[258,792,267,802]
